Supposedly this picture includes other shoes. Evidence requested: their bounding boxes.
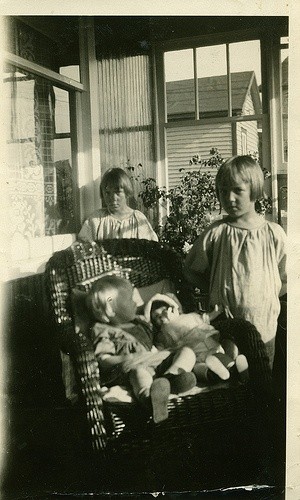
[149,378,169,421]
[234,354,249,380]
[205,356,229,382]
[168,371,196,394]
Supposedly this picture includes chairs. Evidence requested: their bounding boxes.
[46,238,272,468]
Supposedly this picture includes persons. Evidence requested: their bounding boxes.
[184,154,286,422]
[76,167,160,243]
[143,291,249,387]
[87,274,197,425]
